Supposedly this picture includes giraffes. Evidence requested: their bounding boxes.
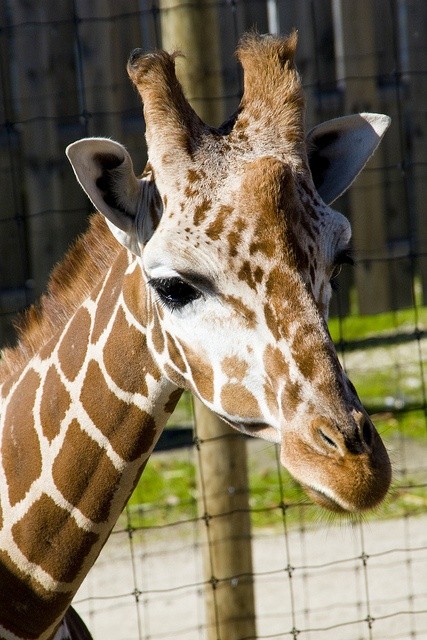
[0,25,407,640]
